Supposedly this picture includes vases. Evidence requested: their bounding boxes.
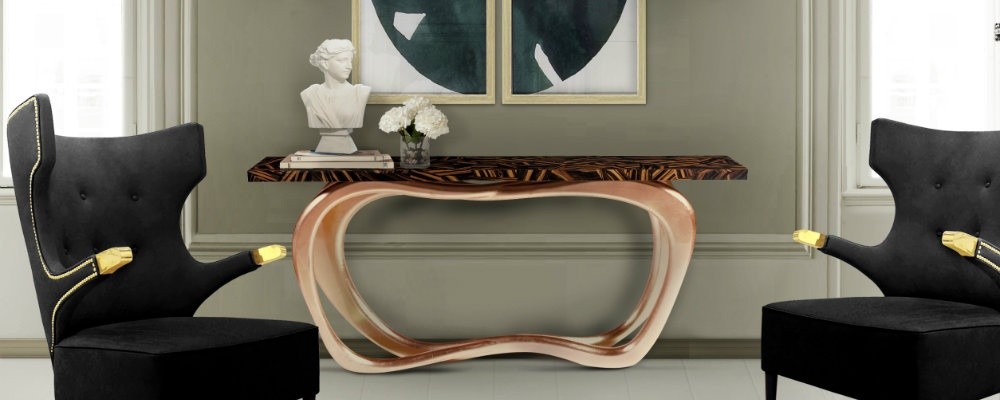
[400,136,431,169]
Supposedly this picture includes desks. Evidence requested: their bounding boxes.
[246,153,749,373]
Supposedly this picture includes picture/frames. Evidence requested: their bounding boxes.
[501,0,648,104]
[352,0,497,105]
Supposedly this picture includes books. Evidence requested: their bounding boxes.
[291,149,392,162]
[280,154,394,169]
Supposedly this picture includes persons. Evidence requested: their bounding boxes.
[299,39,373,129]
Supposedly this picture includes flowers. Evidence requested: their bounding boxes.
[378,99,448,162]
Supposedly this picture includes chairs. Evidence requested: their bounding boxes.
[7,95,322,400]
[761,120,1000,400]
